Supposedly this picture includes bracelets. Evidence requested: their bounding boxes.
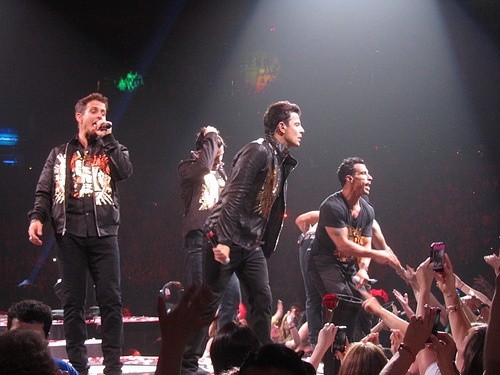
[460,283,465,290]
[397,343,415,362]
[290,323,295,329]
[447,302,463,311]
[30,219,37,223]
[396,268,405,275]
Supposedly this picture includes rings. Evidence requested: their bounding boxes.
[29,236,32,241]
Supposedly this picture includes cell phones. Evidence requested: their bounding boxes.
[331,326,347,353]
[425,307,442,344]
[430,242,446,271]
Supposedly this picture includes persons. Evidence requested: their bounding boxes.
[155,157,500,375]
[175,125,241,330]
[27,92,124,375]
[181,101,305,375]
[0,300,79,375]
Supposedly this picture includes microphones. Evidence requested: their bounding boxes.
[203,224,230,264]
[352,277,371,290]
[99,122,112,131]
